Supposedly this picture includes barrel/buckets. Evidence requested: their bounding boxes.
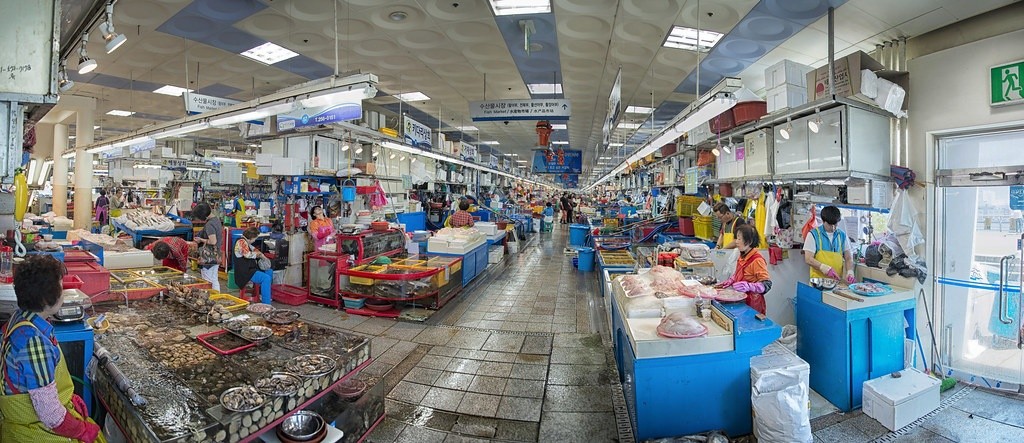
[342,179,355,201]
[507,242,518,254]
[569,226,589,246]
[577,247,595,271]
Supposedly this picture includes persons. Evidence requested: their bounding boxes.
[193,201,223,294]
[449,199,474,228]
[713,202,746,249]
[627,196,634,207]
[95,190,110,226]
[558,193,577,224]
[126,191,134,209]
[447,196,467,215]
[224,191,246,228]
[714,223,772,316]
[802,206,857,285]
[0,253,106,443]
[234,227,273,304]
[143,236,188,273]
[309,207,337,252]
[540,202,553,232]
[444,210,455,227]
[109,188,126,238]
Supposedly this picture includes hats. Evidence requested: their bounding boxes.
[562,191,569,195]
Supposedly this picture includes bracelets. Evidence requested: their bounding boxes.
[716,245,720,249]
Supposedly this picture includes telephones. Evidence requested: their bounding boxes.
[15,174,28,222]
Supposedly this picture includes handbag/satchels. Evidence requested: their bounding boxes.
[258,257,271,271]
[197,239,222,266]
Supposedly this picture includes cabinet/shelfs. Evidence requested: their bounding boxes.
[244,174,410,233]
[654,93,891,189]
[587,217,781,441]
[340,251,461,317]
[796,282,916,413]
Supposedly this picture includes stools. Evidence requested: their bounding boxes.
[239,280,260,303]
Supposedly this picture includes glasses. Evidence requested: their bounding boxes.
[717,214,724,219]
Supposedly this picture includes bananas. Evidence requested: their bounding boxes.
[14,168,28,221]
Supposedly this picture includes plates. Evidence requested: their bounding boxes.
[286,354,336,377]
[714,288,747,301]
[849,283,893,296]
[657,325,708,338]
[262,309,300,324]
[254,372,304,397]
[241,326,272,339]
[678,285,716,299]
[219,387,266,412]
[246,303,274,314]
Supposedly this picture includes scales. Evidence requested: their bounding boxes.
[47,288,92,322]
[241,217,264,224]
[339,228,362,235]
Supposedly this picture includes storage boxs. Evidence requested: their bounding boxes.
[20,216,153,267]
[676,50,910,250]
[428,225,520,264]
[272,282,307,304]
[245,152,422,227]
[862,370,942,431]
[342,253,463,309]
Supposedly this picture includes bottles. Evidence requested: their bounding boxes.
[0,246,13,284]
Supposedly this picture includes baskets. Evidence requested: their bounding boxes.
[357,185,376,194]
[372,222,388,231]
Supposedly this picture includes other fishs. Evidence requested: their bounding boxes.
[344,268,434,297]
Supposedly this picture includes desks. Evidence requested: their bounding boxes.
[50,319,93,418]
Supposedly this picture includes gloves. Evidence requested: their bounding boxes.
[820,263,840,280]
[317,228,332,240]
[714,273,736,288]
[266,253,276,260]
[51,409,99,443]
[71,393,89,420]
[846,270,856,285]
[732,281,765,293]
[227,211,235,217]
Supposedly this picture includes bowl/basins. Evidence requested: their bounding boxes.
[431,203,443,208]
[364,299,395,311]
[371,222,389,232]
[276,411,326,443]
[42,234,53,242]
[809,277,840,291]
[87,318,110,333]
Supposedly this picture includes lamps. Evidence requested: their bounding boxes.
[501,0,823,191]
[76,34,98,74]
[57,55,74,92]
[99,4,127,53]
[44,72,499,171]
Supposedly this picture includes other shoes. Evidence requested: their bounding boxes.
[563,221,566,224]
[560,219,562,224]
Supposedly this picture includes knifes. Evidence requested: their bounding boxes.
[836,275,850,286]
